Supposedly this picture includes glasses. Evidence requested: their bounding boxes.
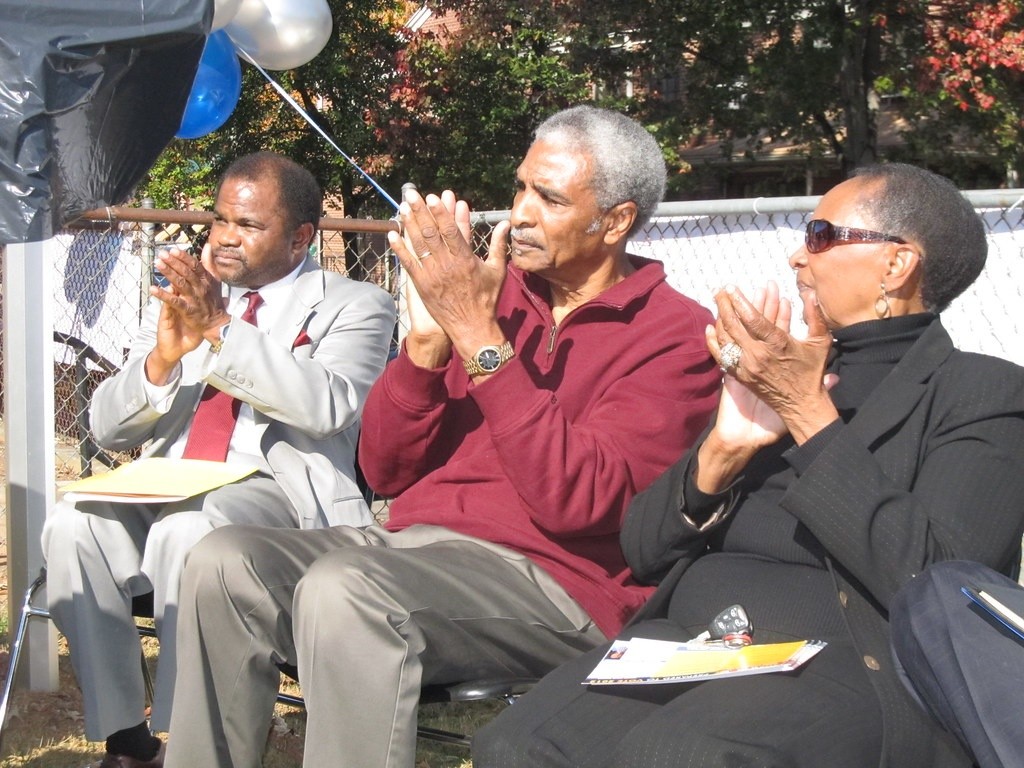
[805,220,920,263]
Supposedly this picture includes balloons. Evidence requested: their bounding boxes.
[173,0,333,139]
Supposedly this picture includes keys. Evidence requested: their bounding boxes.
[687,602,754,648]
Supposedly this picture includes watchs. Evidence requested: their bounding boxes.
[461,341,516,376]
[209,321,231,353]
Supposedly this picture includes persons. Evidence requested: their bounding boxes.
[471,162,1023,768]
[888,558,1024,768]
[40,152,397,768]
[162,104,725,768]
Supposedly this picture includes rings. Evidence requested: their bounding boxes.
[418,251,431,259]
[717,342,743,374]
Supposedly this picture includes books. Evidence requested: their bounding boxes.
[961,576,1024,642]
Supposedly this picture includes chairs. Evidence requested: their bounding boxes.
[275,662,542,746]
[0,565,161,743]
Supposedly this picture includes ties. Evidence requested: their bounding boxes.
[181,291,266,463]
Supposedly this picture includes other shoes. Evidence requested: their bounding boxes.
[100,737,165,768]
